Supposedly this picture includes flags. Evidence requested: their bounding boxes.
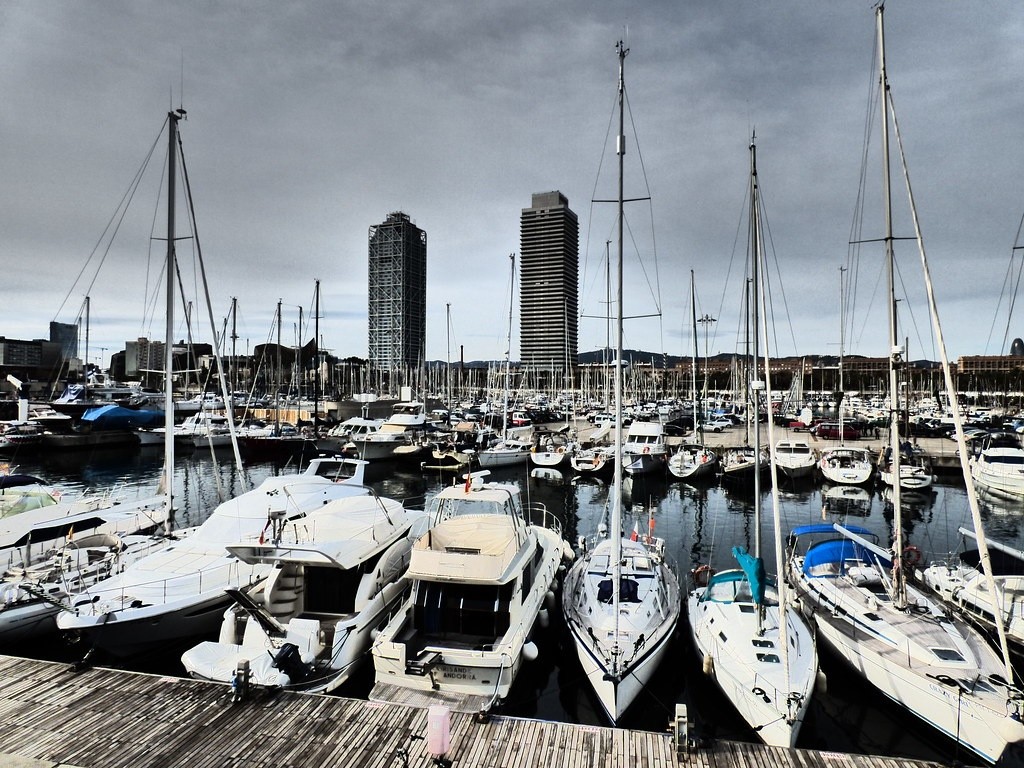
[466,470,472,492]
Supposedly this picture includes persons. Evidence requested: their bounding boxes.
[882,444,892,474]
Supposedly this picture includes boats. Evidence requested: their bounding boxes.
[367,476,573,712]
[1,465,205,653]
[182,493,417,694]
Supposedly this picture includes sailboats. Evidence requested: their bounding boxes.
[908,215,1024,640]
[55,35,368,668]
[780,0,1024,768]
[2,242,1024,520]
[687,126,820,749]
[562,30,679,719]
